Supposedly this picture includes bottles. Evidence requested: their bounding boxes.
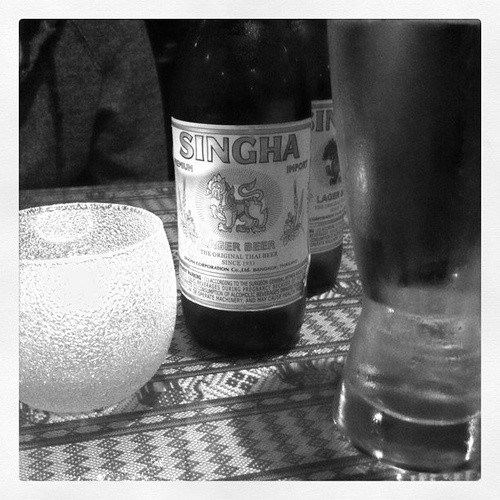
[292,19,346,295]
[172,19,315,356]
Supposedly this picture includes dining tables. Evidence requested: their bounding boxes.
[20,177,480,481]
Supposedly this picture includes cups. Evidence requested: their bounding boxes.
[321,20,482,475]
[21,200,177,413]
